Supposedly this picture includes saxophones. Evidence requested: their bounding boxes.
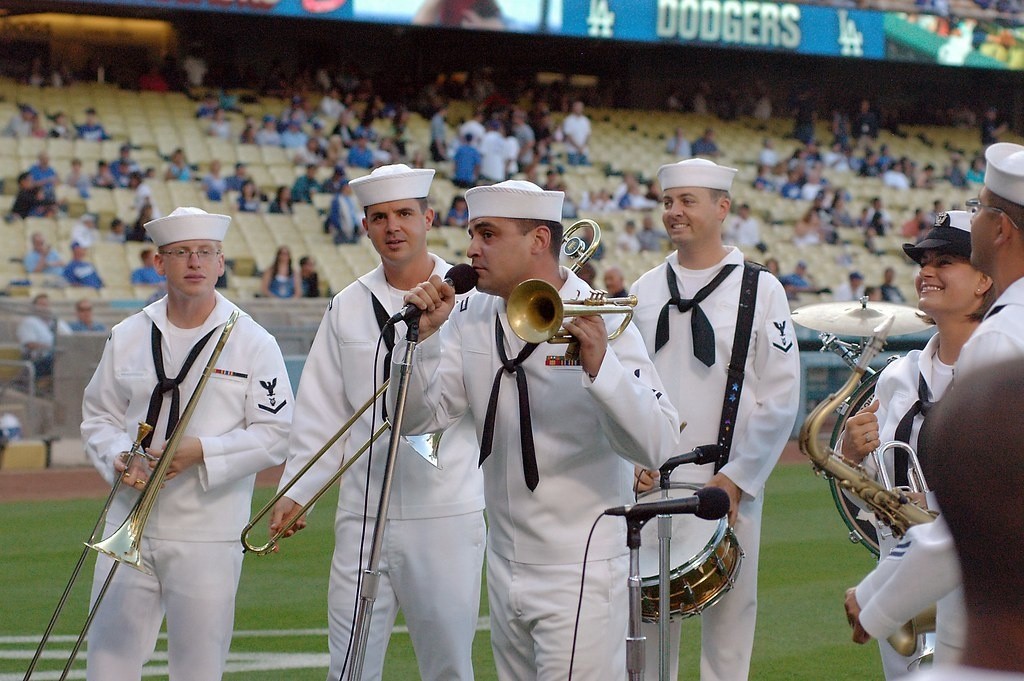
[795,311,941,658]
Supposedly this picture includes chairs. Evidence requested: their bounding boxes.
[0,80,1024,485]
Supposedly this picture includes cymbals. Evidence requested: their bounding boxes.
[790,295,936,338]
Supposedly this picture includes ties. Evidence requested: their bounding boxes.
[986,304,1006,319]
[655,262,738,367]
[371,293,390,430]
[478,311,540,492]
[141,320,218,452]
[892,370,943,536]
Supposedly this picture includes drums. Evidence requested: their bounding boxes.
[638,482,746,627]
[825,354,903,557]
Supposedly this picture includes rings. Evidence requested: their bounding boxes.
[866,433,870,442]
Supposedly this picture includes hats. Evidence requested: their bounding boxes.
[902,208,977,264]
[657,157,738,192]
[464,180,565,222]
[983,142,1024,208]
[143,206,232,247]
[348,162,437,207]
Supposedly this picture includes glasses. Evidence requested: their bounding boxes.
[158,249,221,263]
[963,198,1020,231]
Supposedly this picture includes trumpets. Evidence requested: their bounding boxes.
[505,275,641,366]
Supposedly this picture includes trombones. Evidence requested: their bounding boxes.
[239,217,601,554]
[23,307,244,681]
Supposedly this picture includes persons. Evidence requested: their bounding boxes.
[386,180,681,681]
[0,45,1024,298]
[82,205,295,680]
[270,163,488,681]
[629,158,800,681]
[833,141,1024,681]
[10,295,111,394]
